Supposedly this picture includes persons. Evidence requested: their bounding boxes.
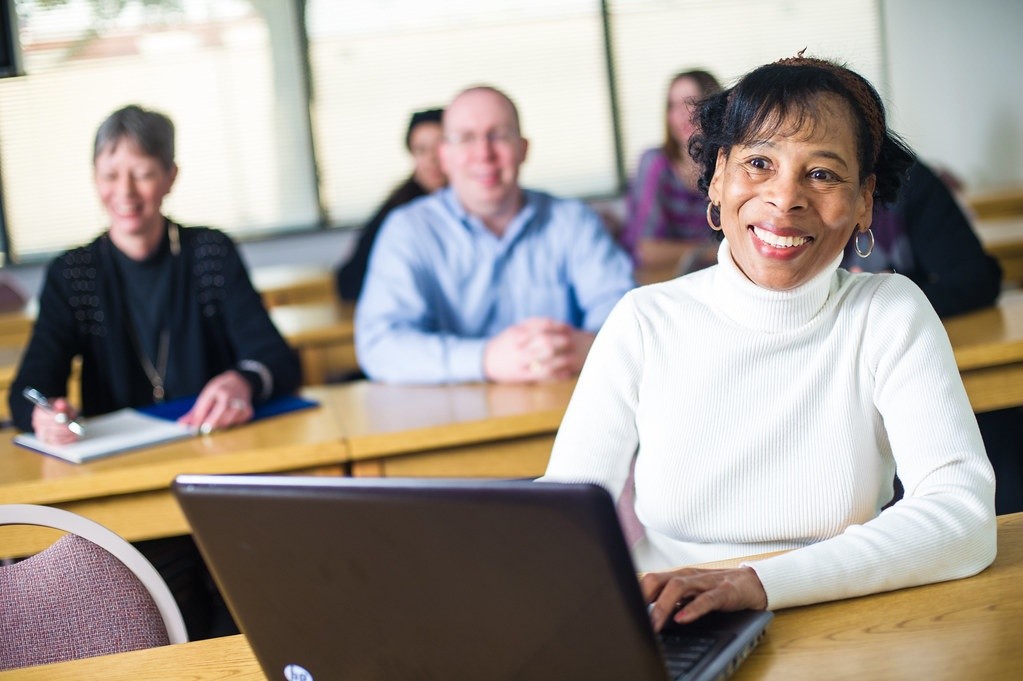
[840,134,1004,318]
[615,70,725,263]
[350,86,637,386]
[531,58,998,631]
[7,105,302,435]
[331,110,449,304]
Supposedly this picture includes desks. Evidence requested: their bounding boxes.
[0,185,1023,681]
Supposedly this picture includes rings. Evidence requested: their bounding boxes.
[229,396,245,411]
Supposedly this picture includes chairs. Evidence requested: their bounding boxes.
[0,504,188,671]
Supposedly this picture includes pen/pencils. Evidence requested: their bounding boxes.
[22,387,84,436]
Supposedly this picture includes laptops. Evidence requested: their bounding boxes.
[172,472,774,681]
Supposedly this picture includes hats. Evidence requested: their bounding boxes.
[406,107,445,149]
